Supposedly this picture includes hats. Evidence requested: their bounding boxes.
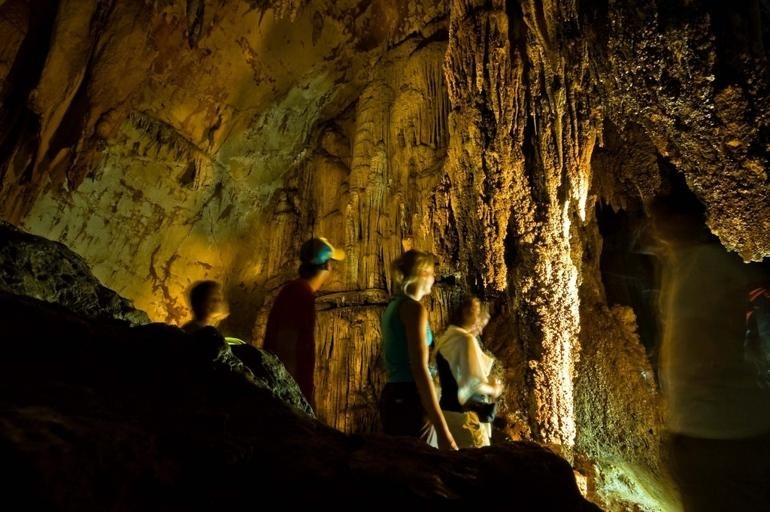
[299,236,347,264]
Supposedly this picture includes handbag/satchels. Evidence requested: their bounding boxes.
[461,397,496,422]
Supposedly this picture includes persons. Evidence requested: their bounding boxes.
[262,237,345,417]
[435,291,504,449]
[381,248,459,449]
[179,280,229,333]
